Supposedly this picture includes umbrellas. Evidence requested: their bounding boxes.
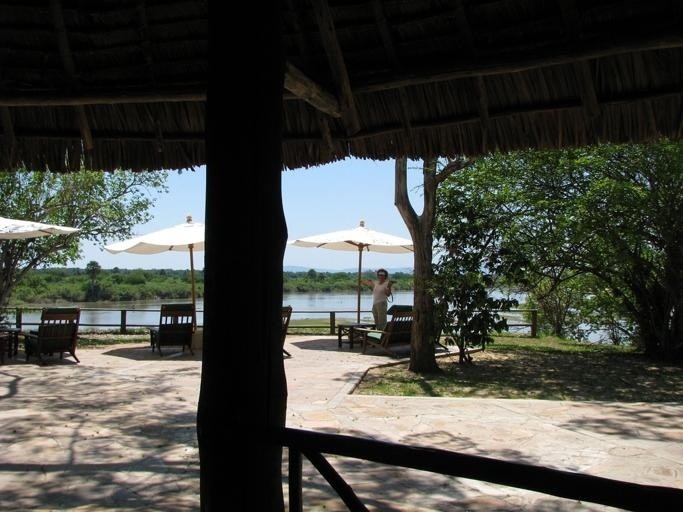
[101,215,205,332]
[285,218,414,328]
[0,216,81,239]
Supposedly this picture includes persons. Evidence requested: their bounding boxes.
[361,269,393,331]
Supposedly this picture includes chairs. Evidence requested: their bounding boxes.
[352,303,450,359]
[149,303,195,357]
[281,306,292,358]
[24,307,82,365]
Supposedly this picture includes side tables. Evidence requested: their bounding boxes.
[339,324,375,349]
[0,327,21,359]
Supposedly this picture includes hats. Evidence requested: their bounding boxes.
[378,271,385,275]
